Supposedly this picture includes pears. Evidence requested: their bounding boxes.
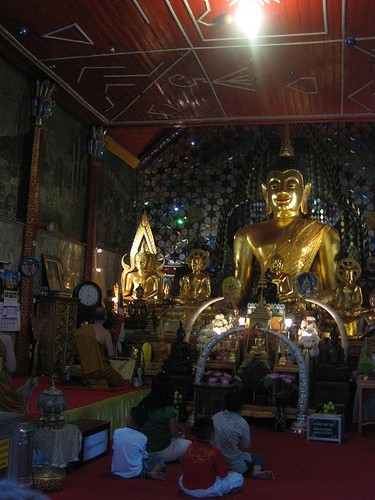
[174,391,182,405]
[323,400,335,413]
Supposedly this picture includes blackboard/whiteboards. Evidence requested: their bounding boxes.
[307,416,341,444]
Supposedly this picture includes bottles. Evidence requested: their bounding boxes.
[133,359,143,387]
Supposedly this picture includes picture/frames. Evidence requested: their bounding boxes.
[42,253,65,292]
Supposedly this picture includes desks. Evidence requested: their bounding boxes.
[357,379,375,435]
[206,360,236,375]
[273,364,300,406]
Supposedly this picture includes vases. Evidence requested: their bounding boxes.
[274,391,289,432]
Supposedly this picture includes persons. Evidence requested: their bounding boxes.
[271,253,293,299]
[110,407,165,480]
[138,371,192,464]
[178,416,243,497]
[333,266,363,322]
[122,251,159,302]
[250,331,265,353]
[210,390,275,480]
[128,284,149,317]
[71,309,124,386]
[233,153,341,306]
[163,326,190,374]
[0,333,36,412]
[171,256,211,306]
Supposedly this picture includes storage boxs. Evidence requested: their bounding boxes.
[0,410,23,482]
[306,414,344,445]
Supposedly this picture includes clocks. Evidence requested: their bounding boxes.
[18,256,41,278]
[73,280,102,310]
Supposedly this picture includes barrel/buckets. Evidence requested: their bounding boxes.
[8,423,33,487]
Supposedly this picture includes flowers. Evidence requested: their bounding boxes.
[198,369,243,388]
[258,371,296,398]
